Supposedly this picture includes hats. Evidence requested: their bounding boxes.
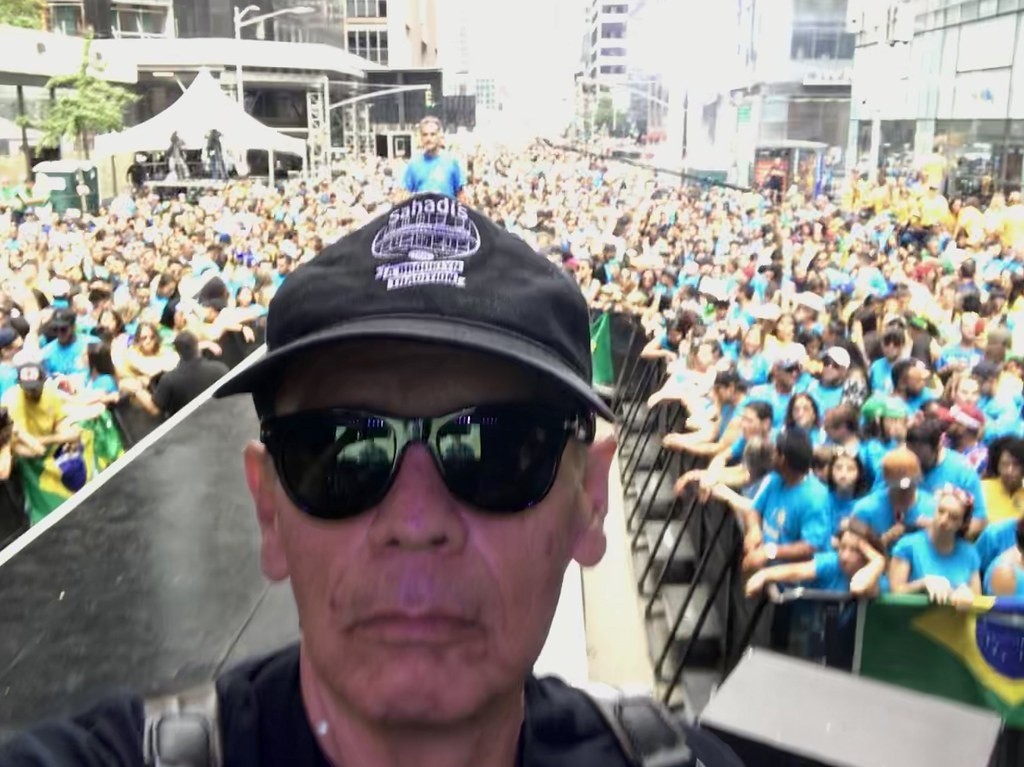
[882,326,906,345]
[792,291,826,313]
[18,364,43,387]
[884,451,920,486]
[211,191,616,422]
[49,309,75,326]
[0,328,14,348]
[821,347,850,366]
[778,358,798,369]
[220,233,232,242]
[90,282,112,292]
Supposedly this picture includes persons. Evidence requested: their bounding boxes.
[0,116,1023,672]
[0,192,746,767]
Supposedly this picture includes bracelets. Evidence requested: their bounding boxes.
[765,543,778,560]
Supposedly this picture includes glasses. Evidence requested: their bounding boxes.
[786,367,798,372]
[259,401,595,519]
[885,335,901,346]
[823,360,839,368]
[53,327,67,332]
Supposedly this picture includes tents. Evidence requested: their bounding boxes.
[93,64,309,194]
